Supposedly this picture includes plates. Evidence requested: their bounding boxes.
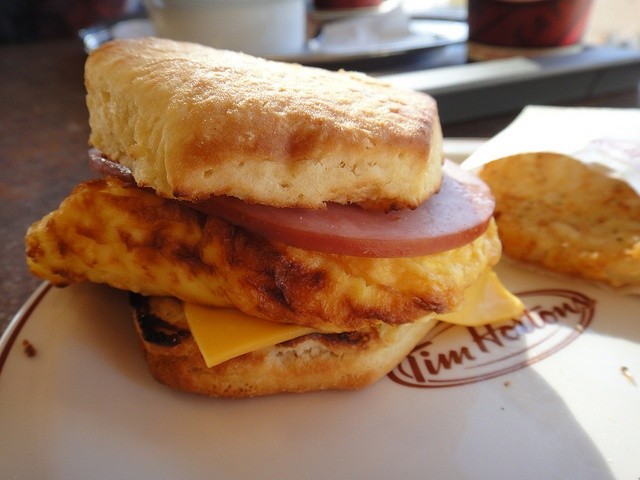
[302,18,465,63]
[0,136,640,475]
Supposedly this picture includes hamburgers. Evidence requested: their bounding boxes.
[25,37,524,397]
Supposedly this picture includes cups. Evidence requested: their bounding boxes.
[148,2,308,57]
[470,0,587,46]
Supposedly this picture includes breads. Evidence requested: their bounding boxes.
[483,152,640,291]
